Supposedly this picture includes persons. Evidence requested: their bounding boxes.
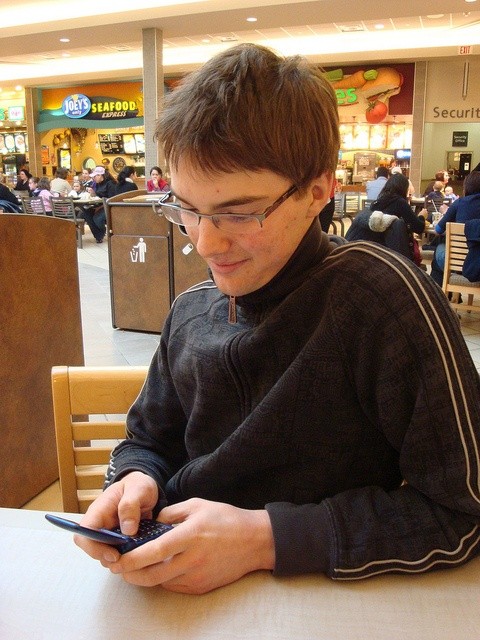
[319,173,337,233]
[431,162,480,287]
[73,43,480,596]
[114,166,138,195]
[28,177,40,196]
[82,168,97,191]
[84,167,117,242]
[14,169,33,190]
[31,176,60,216]
[50,167,72,196]
[0,183,20,209]
[147,166,170,192]
[424,170,449,198]
[367,166,389,202]
[370,173,428,232]
[426,181,445,221]
[445,187,458,200]
[67,180,87,197]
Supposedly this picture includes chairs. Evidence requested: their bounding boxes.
[425,198,453,215]
[331,195,344,237]
[50,366,148,513]
[442,222,480,314]
[337,192,361,225]
[10,189,28,196]
[362,200,375,209]
[421,264,427,270]
[49,196,85,249]
[21,197,46,216]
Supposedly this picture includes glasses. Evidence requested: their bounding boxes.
[158,186,297,234]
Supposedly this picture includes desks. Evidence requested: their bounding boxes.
[1,508,479,640]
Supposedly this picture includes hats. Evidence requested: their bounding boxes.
[89,167,105,177]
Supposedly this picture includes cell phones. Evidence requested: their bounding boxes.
[43,512,175,556]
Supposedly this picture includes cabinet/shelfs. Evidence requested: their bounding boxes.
[105,188,210,333]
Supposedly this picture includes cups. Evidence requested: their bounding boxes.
[432,212,443,227]
